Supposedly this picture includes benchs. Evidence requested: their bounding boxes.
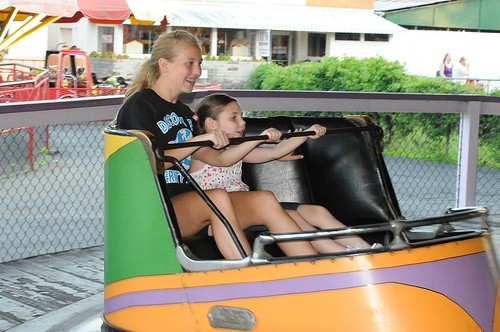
[111,116,409,259]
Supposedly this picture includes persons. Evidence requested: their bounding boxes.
[188,93,382,257]
[440,54,454,78]
[115,30,318,260]
[456,57,470,77]
